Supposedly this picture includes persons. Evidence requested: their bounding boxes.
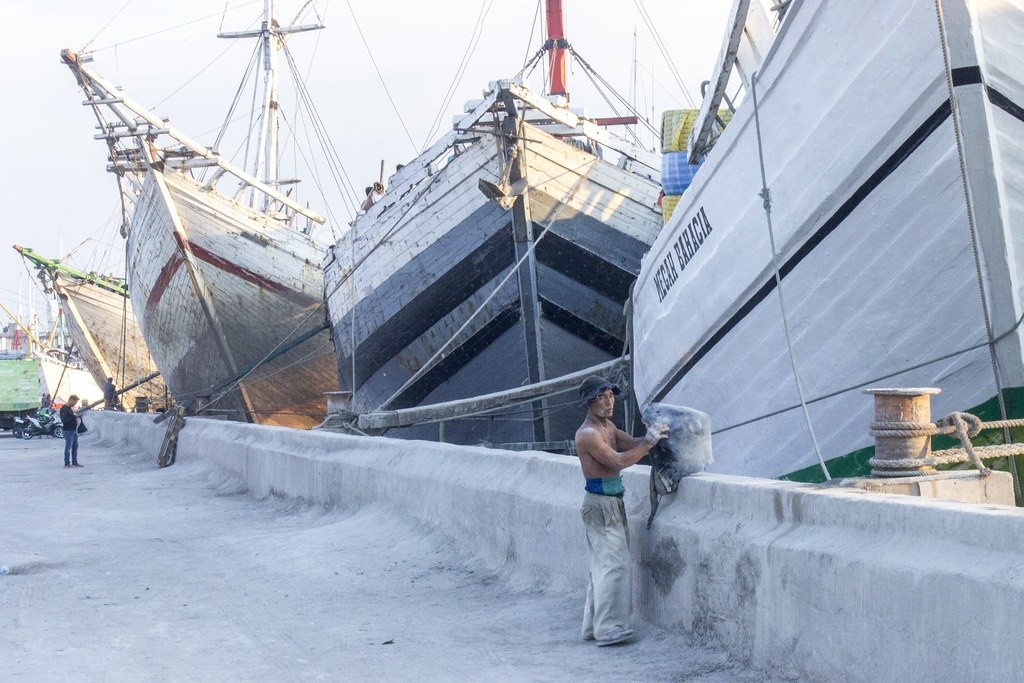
[360,187,375,214]
[41,392,52,408]
[104,378,115,404]
[397,164,413,199]
[60,393,81,467]
[575,375,670,648]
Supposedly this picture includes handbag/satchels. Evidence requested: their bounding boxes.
[78,417,87,433]
[641,402,714,530]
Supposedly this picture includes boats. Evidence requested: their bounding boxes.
[2,0,1023,509]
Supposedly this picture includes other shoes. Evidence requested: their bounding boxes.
[595,628,637,647]
[64,464,76,468]
[72,463,83,467]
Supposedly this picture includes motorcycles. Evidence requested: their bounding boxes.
[13,409,64,440]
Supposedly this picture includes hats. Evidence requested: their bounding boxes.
[578,375,622,408]
